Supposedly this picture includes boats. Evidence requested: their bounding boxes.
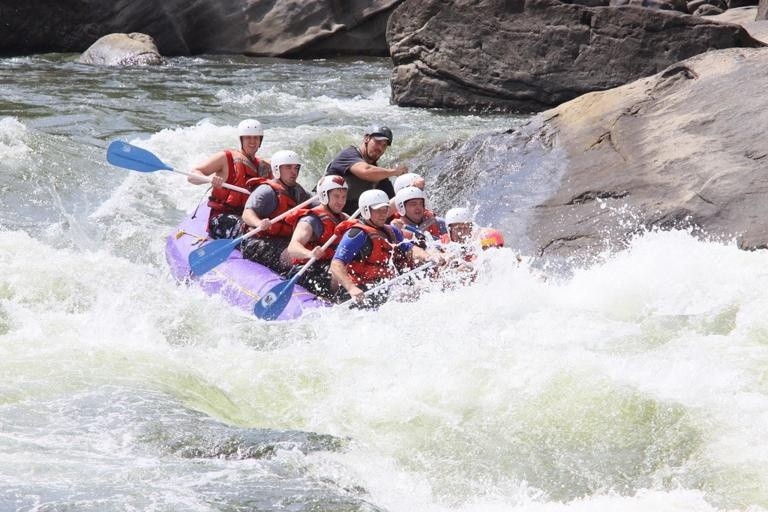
[165,198,508,333]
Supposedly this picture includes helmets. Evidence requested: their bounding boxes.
[363,124,392,146]
[394,172,425,195]
[358,189,390,221]
[394,187,426,217]
[238,118,263,137]
[445,208,472,237]
[317,175,349,206]
[270,150,301,179]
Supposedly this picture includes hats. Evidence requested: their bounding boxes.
[374,136,391,145]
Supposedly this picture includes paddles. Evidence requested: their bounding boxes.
[254,209,362,321]
[106,140,251,195]
[189,193,321,277]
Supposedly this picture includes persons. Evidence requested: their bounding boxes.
[280,124,463,303]
[241,149,312,239]
[187,119,275,241]
